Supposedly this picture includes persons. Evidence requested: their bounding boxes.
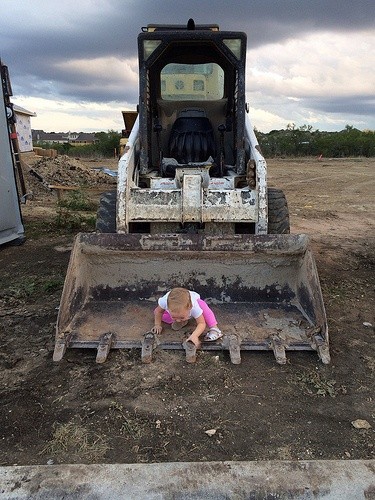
[151,287,222,346]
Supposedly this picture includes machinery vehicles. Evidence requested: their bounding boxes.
[51,17,333,364]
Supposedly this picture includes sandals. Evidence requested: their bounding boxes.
[203,328,223,341]
[171,320,189,330]
